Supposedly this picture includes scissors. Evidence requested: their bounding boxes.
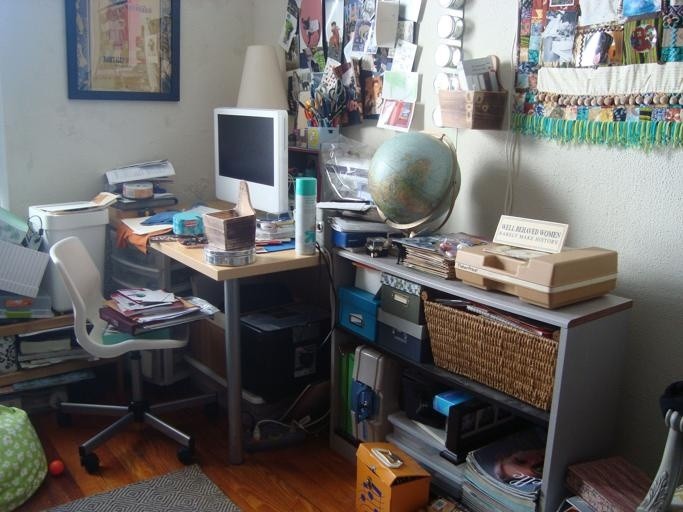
[329,86,346,111]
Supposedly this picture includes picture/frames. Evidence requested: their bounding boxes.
[66,1,180,101]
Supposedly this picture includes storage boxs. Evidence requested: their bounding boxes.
[338,262,433,441]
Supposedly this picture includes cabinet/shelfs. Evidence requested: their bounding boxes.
[0,313,125,402]
[330,247,634,512]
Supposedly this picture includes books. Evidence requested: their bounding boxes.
[391,231,491,280]
[17,334,92,369]
[461,426,547,512]
[110,196,178,211]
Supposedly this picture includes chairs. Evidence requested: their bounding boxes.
[50,235,218,472]
[557,379,683,512]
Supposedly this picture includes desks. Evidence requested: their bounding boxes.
[108,200,337,464]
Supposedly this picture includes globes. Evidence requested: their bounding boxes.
[368,130,460,265]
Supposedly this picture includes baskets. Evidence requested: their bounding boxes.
[422,298,559,413]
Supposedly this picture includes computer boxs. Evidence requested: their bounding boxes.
[240,302,332,404]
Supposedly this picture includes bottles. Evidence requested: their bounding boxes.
[293,178,318,256]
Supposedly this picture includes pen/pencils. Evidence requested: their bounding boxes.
[298,84,346,127]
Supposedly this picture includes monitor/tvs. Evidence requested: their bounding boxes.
[213,107,288,217]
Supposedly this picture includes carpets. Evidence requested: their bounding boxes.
[38,463,241,512]
